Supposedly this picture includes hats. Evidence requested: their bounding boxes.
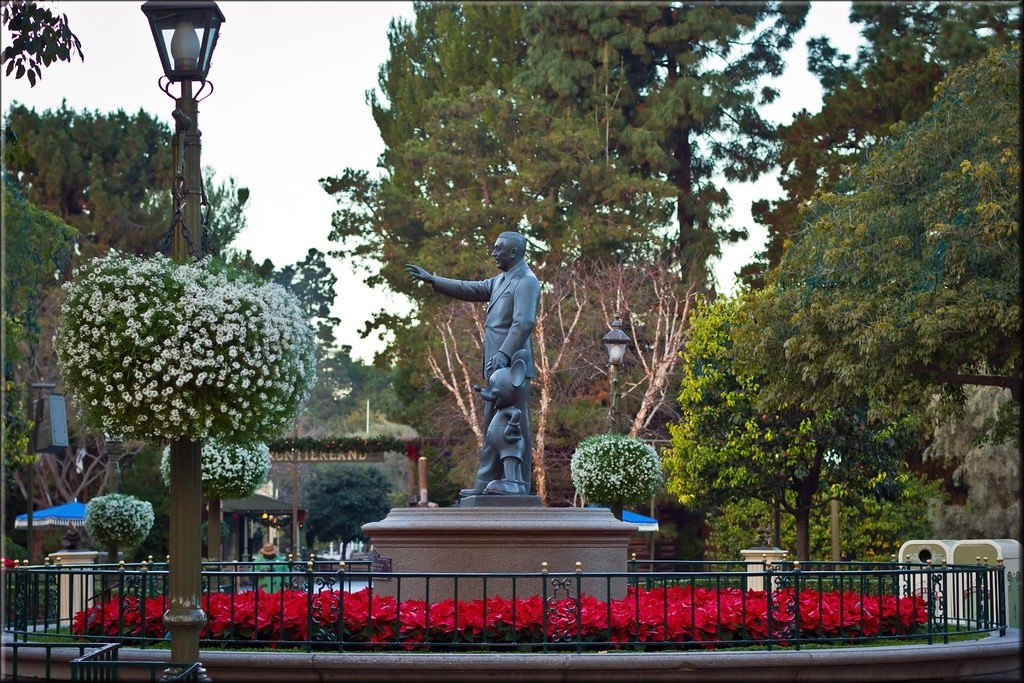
[260,543,277,555]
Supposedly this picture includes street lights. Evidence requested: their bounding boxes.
[105,431,125,564]
[824,449,841,573]
[601,311,631,519]
[141,0,227,683]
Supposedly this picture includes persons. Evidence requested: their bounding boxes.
[403,232,541,495]
[253,545,290,597]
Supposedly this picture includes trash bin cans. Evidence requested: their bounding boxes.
[898,540,1021,630]
